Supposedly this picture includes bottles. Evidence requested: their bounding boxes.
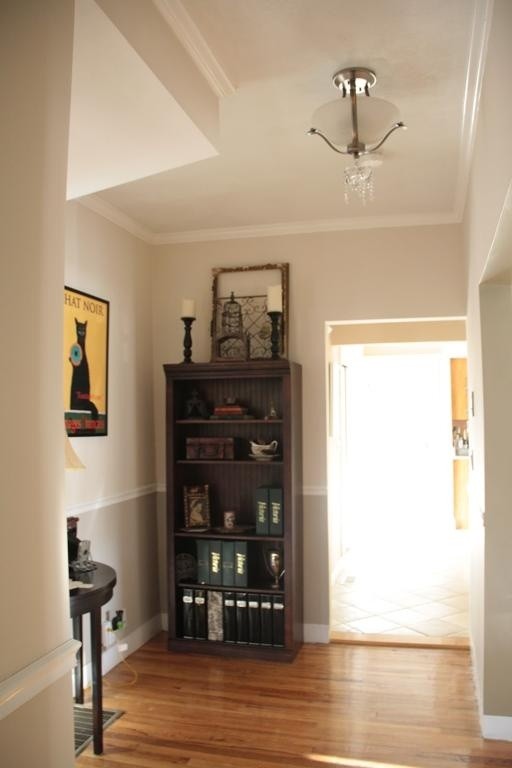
[67,515,81,594]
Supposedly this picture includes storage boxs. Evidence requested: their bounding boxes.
[184,434,237,462]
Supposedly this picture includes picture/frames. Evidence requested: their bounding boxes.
[64,287,113,437]
[208,259,292,359]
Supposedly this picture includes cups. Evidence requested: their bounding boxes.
[223,510,237,528]
[76,540,91,567]
[249,440,279,456]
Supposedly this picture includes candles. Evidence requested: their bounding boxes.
[180,295,198,319]
[264,281,284,314]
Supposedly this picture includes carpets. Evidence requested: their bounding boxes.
[69,703,127,757]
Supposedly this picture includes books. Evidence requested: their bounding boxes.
[176,483,285,647]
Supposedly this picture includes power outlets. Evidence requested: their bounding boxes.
[102,620,116,648]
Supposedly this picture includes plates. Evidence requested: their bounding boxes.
[246,452,278,461]
[212,524,256,534]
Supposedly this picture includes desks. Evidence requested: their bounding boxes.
[68,559,121,758]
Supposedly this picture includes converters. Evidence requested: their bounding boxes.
[111,616,125,633]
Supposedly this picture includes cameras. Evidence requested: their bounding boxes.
[76,538,91,569]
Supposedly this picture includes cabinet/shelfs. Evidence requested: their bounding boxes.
[160,358,305,661]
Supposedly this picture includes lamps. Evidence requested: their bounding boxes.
[308,61,412,209]
